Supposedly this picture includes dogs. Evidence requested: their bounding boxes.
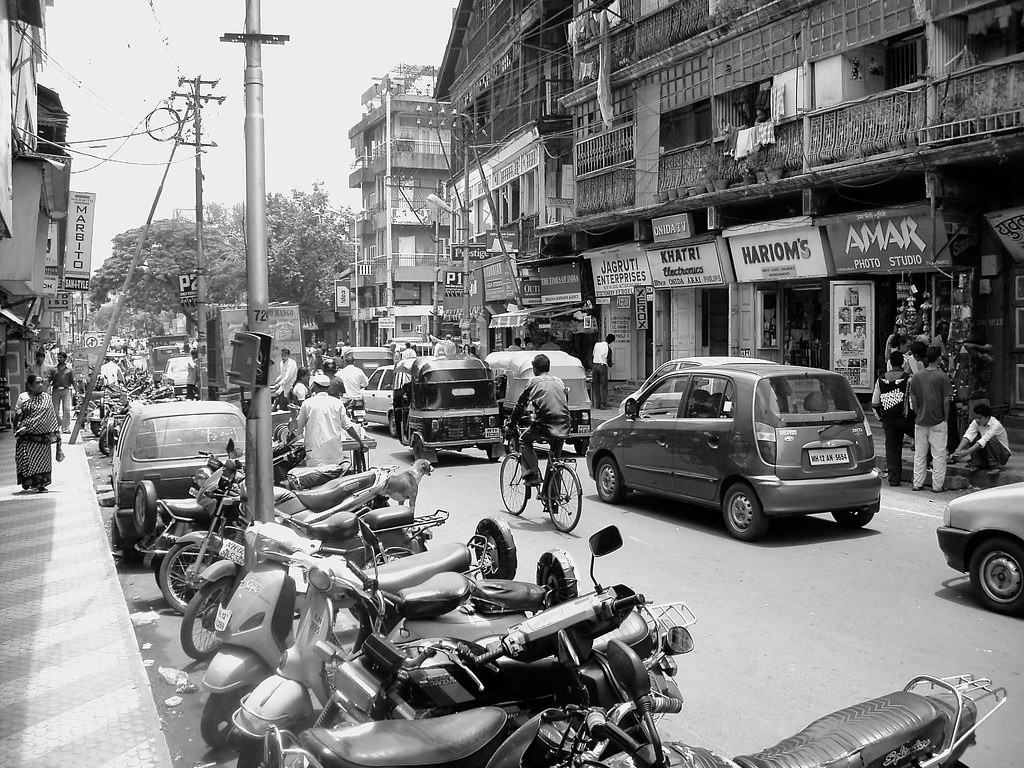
[382,459,435,511]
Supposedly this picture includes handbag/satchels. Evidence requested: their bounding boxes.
[56,439,65,461]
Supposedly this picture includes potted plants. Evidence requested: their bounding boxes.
[760,155,785,181]
[705,0,749,30]
[754,160,767,184]
[737,160,757,186]
[701,152,722,192]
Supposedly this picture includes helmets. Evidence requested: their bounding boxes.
[323,359,336,372]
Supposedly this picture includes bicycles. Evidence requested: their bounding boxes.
[497,420,585,534]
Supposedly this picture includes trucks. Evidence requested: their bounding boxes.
[206,304,308,399]
[146,332,194,396]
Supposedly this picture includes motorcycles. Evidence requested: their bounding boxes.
[74,361,199,454]
[143,437,1008,768]
[390,353,503,464]
[343,347,396,381]
[484,351,592,454]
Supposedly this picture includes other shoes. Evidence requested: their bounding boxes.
[62,427,69,433]
[37,487,48,493]
[547,503,559,514]
[22,484,29,490]
[524,471,544,487]
[987,466,1003,474]
[970,465,986,472]
[596,406,600,408]
[946,457,958,464]
[600,406,610,410]
[913,486,924,491]
[932,486,948,492]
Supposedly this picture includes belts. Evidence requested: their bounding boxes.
[54,386,67,389]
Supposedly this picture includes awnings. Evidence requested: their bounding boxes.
[489,300,587,328]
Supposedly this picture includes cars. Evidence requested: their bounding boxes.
[364,366,400,439]
[106,401,248,553]
[936,482,1024,615]
[584,364,884,544]
[618,356,799,422]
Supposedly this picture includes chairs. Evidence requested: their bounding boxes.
[696,392,729,417]
[803,391,828,413]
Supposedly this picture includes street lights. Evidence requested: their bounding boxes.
[326,206,362,347]
[425,194,471,355]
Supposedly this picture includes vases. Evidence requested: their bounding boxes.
[659,191,668,203]
[687,187,697,196]
[676,186,690,198]
[652,194,660,204]
[712,178,730,191]
[667,189,678,200]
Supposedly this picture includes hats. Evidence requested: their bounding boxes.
[313,375,330,386]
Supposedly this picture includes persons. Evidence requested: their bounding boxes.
[592,334,616,410]
[933,322,968,367]
[839,290,865,350]
[950,404,1012,474]
[13,375,61,493]
[109,337,143,354]
[514,336,576,357]
[101,356,125,385]
[427,333,457,356]
[271,336,368,412]
[294,376,369,468]
[28,343,78,433]
[186,349,199,401]
[507,354,571,508]
[390,342,417,364]
[964,343,995,424]
[871,336,953,491]
